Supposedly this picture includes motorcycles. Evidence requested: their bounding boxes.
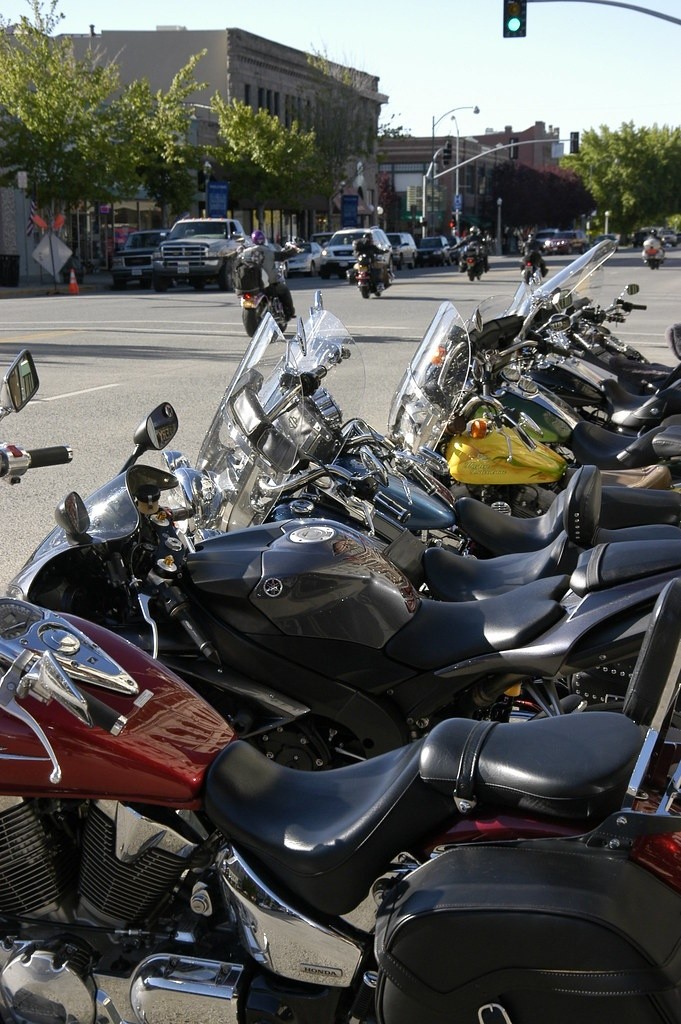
[1,350,680,1024]
[641,237,673,271]
[465,241,488,281]
[522,254,546,287]
[234,288,295,342]
[351,254,396,299]
[192,238,680,586]
[5,397,680,854]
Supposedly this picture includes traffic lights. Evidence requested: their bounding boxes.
[503,0,528,40]
[443,141,454,167]
[448,215,456,227]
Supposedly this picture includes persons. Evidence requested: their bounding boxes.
[236,232,299,319]
[641,229,665,264]
[352,233,392,290]
[520,234,549,277]
[452,230,461,266]
[447,225,491,273]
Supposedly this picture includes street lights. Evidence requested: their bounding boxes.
[495,195,506,258]
[604,210,615,236]
[450,116,462,239]
[204,160,214,220]
[431,104,481,236]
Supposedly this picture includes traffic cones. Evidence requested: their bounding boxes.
[68,268,81,294]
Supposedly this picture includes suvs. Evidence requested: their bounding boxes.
[151,217,250,293]
[418,236,453,266]
[112,230,170,289]
[545,230,591,254]
[319,226,393,281]
[387,232,419,269]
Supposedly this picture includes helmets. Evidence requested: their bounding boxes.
[362,232,373,239]
[251,230,265,245]
[528,234,534,238]
[650,229,658,236]
[469,226,480,236]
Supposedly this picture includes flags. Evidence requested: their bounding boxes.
[26,199,38,236]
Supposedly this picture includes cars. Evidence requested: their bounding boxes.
[283,241,326,279]
[633,228,681,250]
[594,234,620,252]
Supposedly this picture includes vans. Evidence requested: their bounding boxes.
[534,228,560,251]
